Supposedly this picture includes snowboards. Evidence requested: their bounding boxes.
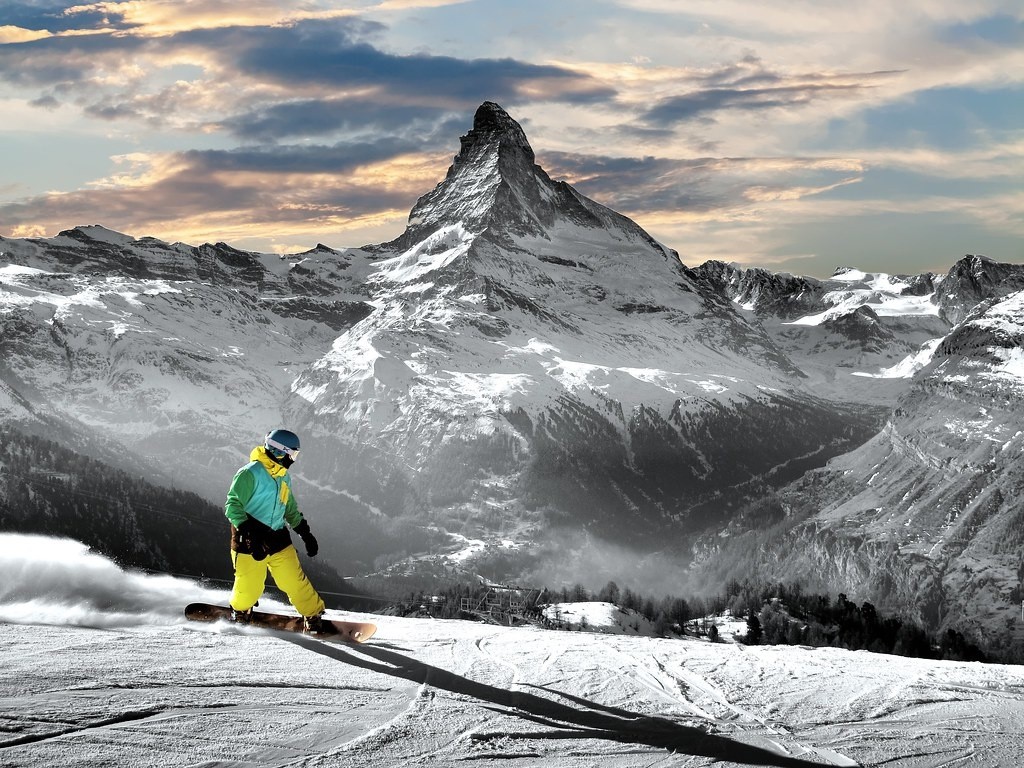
[181,602,379,643]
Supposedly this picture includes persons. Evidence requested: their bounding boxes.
[225,433,323,635]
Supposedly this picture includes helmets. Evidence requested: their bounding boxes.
[265,430,300,461]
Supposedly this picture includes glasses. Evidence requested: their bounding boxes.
[267,438,300,461]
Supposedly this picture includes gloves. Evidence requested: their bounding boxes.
[293,519,319,557]
[238,519,267,561]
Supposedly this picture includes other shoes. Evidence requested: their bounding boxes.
[303,611,322,630]
[230,604,253,624]
